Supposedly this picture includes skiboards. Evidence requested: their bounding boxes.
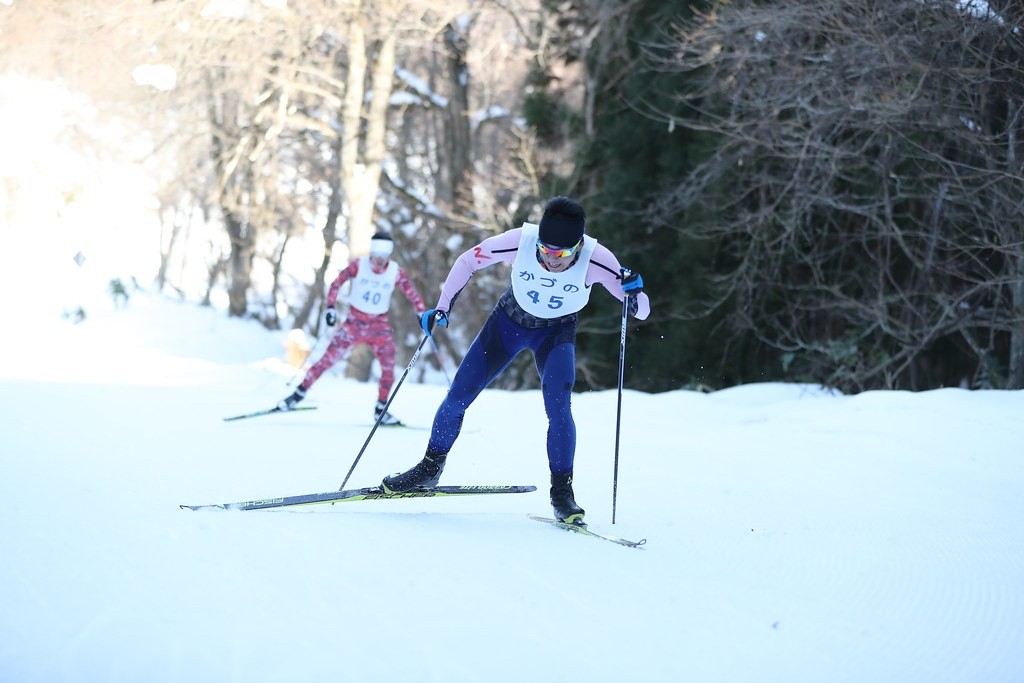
[222,407,433,432]
[178,484,649,549]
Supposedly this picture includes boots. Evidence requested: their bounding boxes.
[277,385,307,411]
[550,472,585,525]
[381,445,448,494]
[374,400,400,424]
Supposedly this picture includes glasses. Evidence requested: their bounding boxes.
[372,251,391,258]
[536,239,582,258]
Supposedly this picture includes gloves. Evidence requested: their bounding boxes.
[417,311,426,329]
[326,306,336,326]
[421,309,448,337]
[616,272,644,295]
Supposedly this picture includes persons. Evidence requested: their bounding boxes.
[380,195,651,529]
[277,231,428,426]
